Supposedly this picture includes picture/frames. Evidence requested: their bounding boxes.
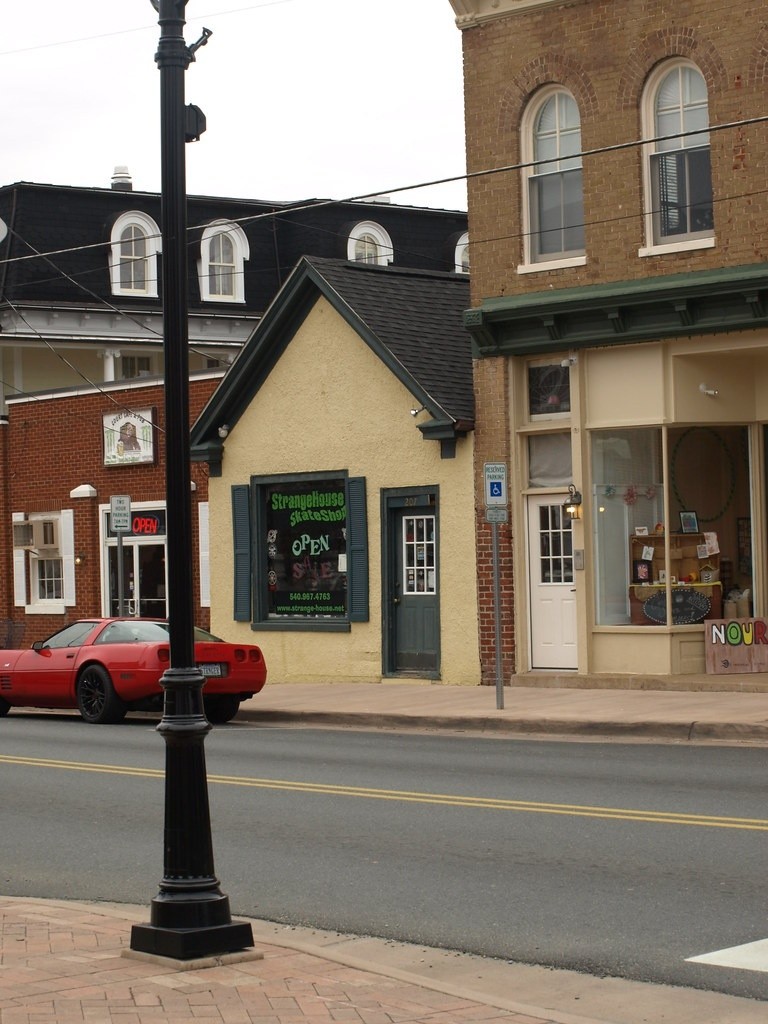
[632,559,654,583]
[678,511,700,534]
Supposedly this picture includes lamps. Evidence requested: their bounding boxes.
[73,549,88,568]
[705,389,719,396]
[70,485,98,499]
[218,425,229,437]
[410,406,426,418]
[564,483,582,520]
[560,359,574,368]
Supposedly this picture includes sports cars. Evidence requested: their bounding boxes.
[0,617,267,724]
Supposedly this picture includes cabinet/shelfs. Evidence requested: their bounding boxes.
[629,534,722,625]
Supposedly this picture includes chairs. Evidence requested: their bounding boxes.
[105,632,134,642]
